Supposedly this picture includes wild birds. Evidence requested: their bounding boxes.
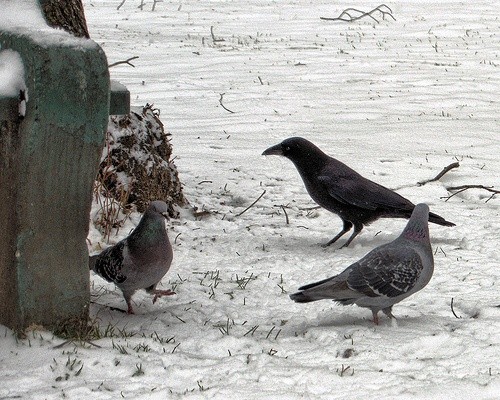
[261,136,457,248]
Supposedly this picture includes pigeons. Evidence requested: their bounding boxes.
[87,201,178,315]
[289,203,435,327]
